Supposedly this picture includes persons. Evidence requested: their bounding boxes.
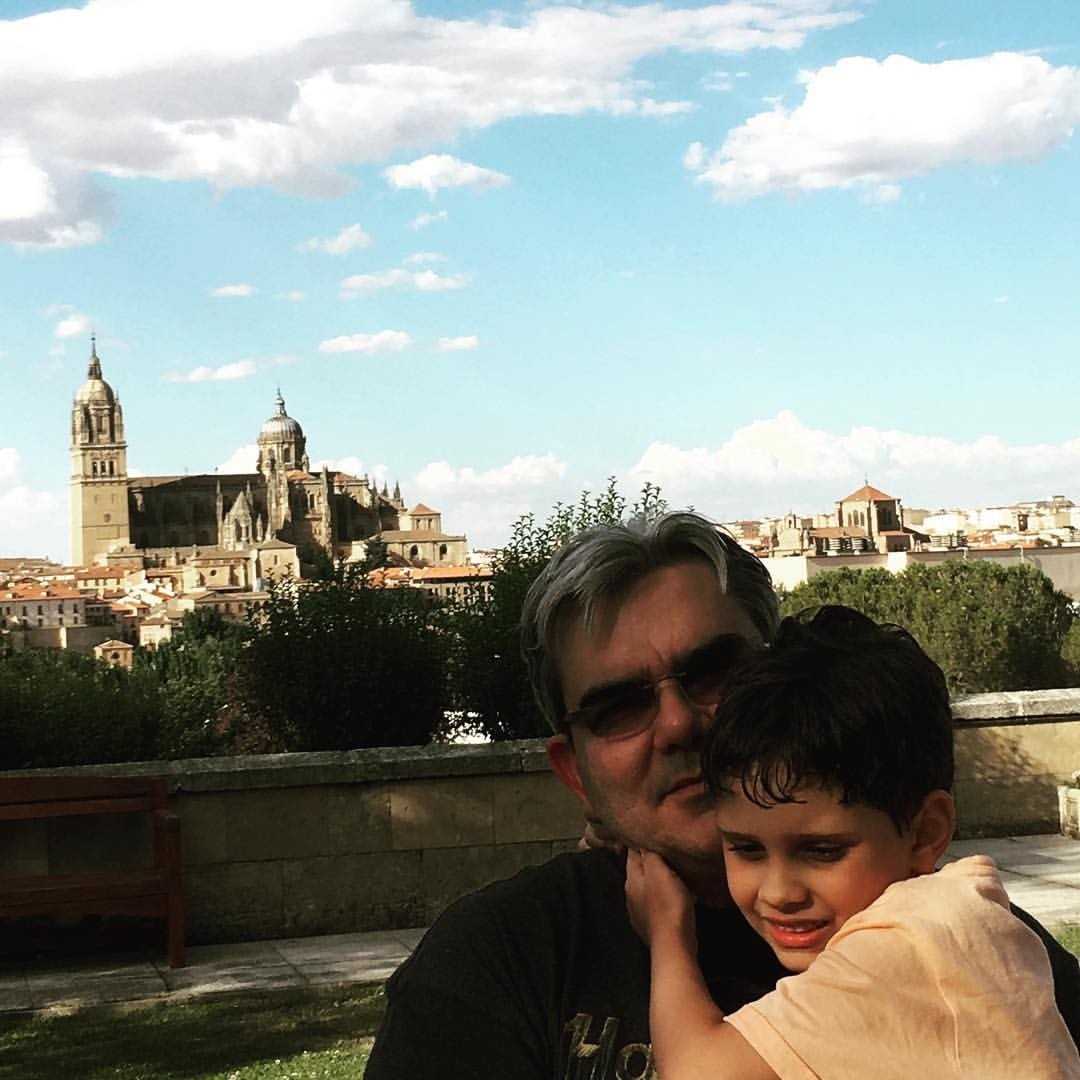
[576,606,1080,1080]
[364,513,1080,1080]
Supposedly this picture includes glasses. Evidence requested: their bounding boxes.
[552,633,772,738]
